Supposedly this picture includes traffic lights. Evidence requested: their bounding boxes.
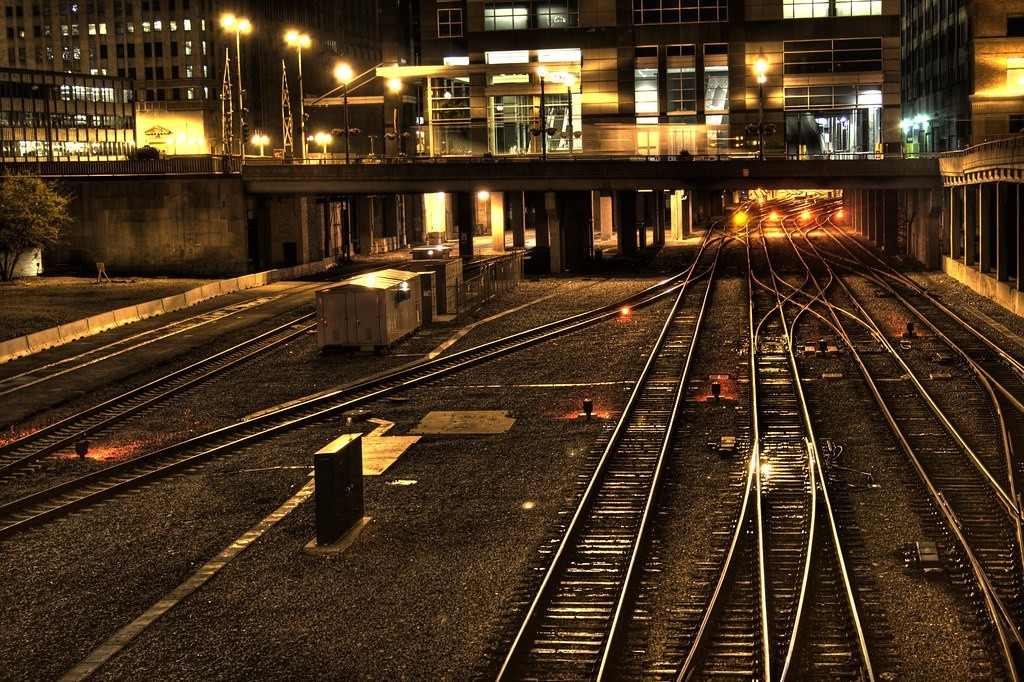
[242,121,250,144]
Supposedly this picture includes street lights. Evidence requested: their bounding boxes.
[218,11,253,166]
[251,134,269,156]
[388,79,403,153]
[561,75,576,155]
[315,131,334,153]
[536,65,549,159]
[334,62,354,165]
[753,53,766,160]
[284,28,313,163]
